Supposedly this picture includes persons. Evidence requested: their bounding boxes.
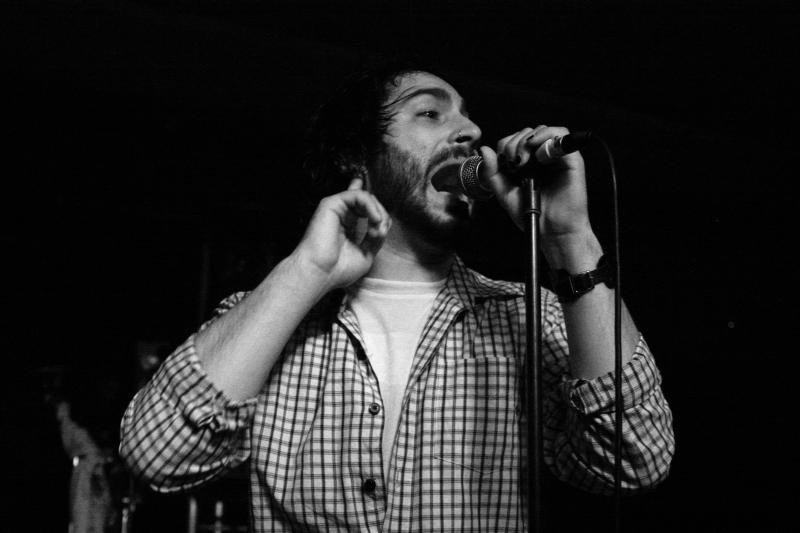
[117,72,676,533]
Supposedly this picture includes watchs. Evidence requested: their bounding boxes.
[554,255,615,304]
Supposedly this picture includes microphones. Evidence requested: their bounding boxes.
[459,132,596,201]
